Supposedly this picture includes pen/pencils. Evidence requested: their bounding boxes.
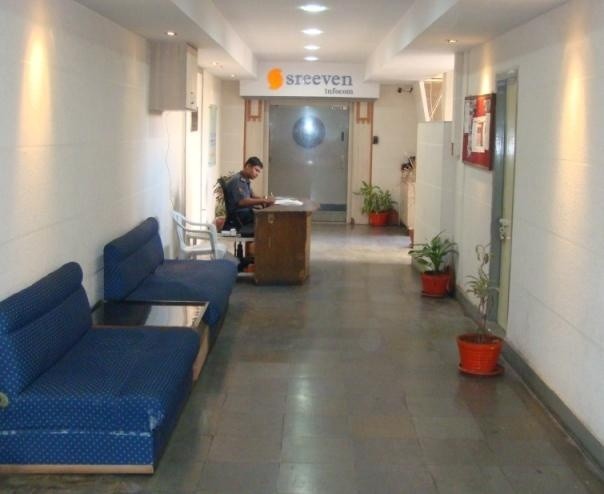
[270,192,273,197]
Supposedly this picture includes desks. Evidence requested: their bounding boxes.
[253,196,320,285]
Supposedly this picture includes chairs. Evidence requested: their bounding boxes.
[171,174,256,272]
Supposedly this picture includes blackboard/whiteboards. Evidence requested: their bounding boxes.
[462,93,495,171]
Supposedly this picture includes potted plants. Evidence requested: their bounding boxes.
[408,233,460,296]
[353,180,392,226]
[456,242,504,377]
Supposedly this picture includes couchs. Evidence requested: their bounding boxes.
[0,262,200,474]
[104,217,236,324]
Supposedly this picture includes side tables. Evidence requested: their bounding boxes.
[91,299,212,383]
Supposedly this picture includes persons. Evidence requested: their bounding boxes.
[227,157,275,254]
